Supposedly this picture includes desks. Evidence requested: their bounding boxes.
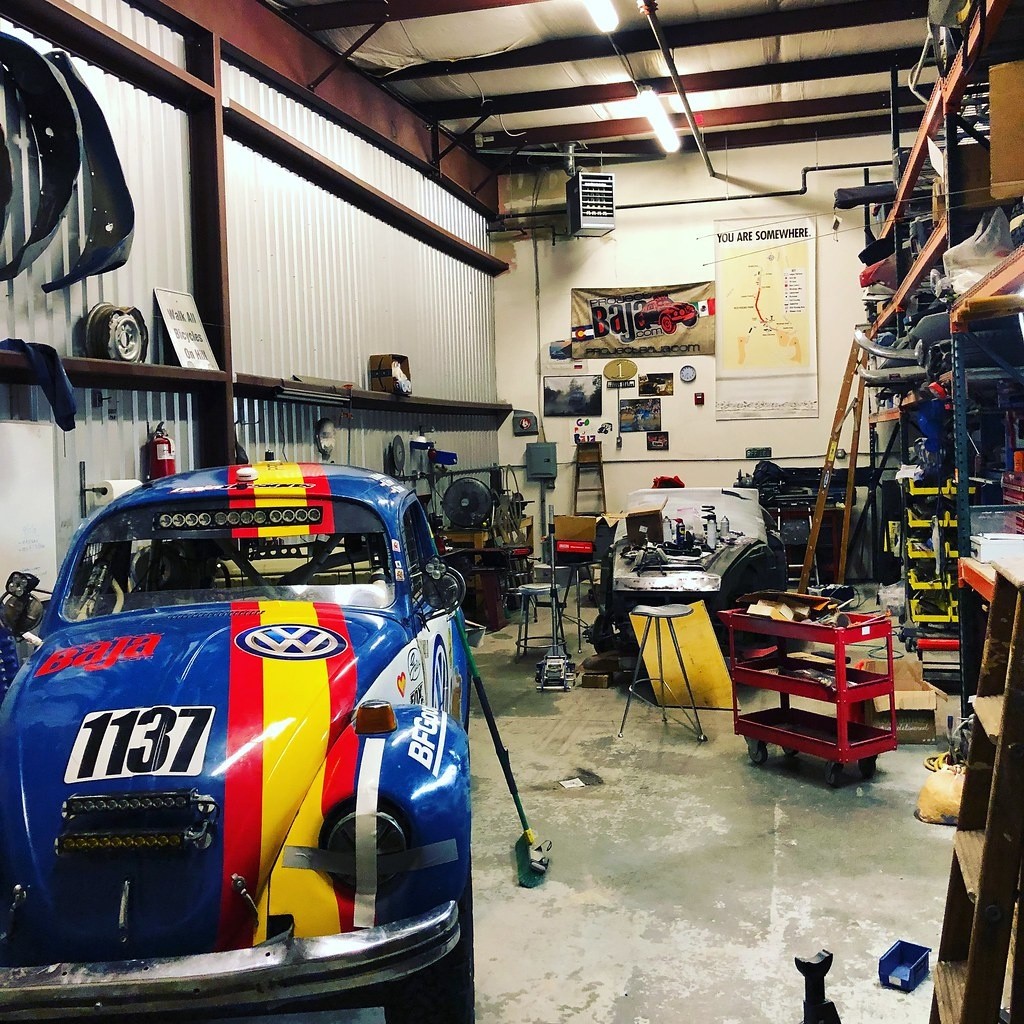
[762,503,841,583]
[611,537,773,686]
[442,516,535,623]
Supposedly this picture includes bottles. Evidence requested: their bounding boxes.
[720,516,729,536]
[663,516,684,546]
[1014,451,1024,472]
[707,520,716,549]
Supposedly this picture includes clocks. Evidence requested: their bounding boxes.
[680,365,696,382]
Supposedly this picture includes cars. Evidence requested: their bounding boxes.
[0,456,481,1024]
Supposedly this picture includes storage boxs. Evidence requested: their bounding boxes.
[863,659,950,744]
[626,495,670,542]
[555,515,597,554]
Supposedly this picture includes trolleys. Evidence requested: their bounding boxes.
[714,602,899,787]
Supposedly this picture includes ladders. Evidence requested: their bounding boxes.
[930,555,1024,1024]
[798,324,872,595]
[572,440,608,522]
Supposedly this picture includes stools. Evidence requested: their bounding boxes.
[515,583,571,665]
[617,604,709,745]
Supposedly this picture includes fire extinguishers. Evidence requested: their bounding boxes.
[150,421,176,480]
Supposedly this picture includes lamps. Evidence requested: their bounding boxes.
[588,0,619,34]
[637,84,683,153]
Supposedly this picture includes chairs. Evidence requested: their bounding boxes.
[775,502,821,588]
[554,551,605,653]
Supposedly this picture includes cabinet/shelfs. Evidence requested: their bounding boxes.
[872,0,1024,764]
[715,608,898,788]
